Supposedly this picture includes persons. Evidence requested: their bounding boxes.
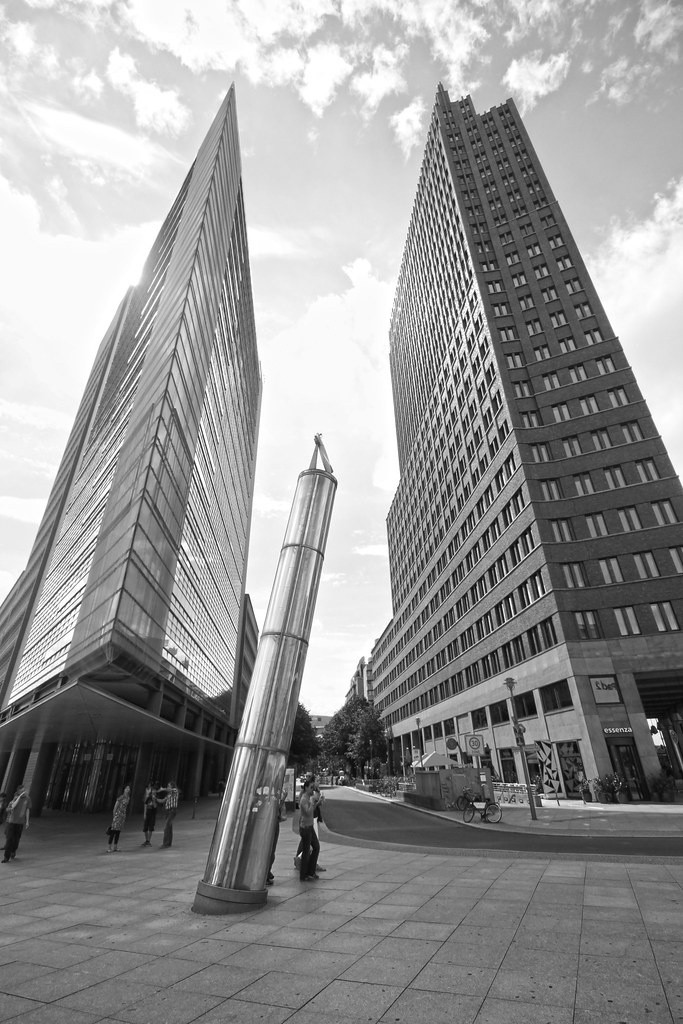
[294,772,325,881]
[266,786,288,888]
[140,780,180,848]
[106,785,131,853]
[0,785,32,864]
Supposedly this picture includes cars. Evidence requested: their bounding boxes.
[296,778,305,808]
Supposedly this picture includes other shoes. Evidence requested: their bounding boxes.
[11,856,14,860]
[312,874,319,879]
[300,876,314,881]
[294,857,301,868]
[114,848,120,852]
[142,841,151,847]
[106,850,111,853]
[268,872,274,879]
[315,864,326,871]
[265,879,273,885]
[1,858,8,863]
[159,845,170,849]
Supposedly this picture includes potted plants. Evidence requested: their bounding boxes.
[579,772,628,804]
[653,769,677,801]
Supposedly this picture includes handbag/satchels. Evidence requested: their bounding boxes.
[106,826,111,835]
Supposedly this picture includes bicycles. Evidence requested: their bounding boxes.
[375,779,399,798]
[456,788,489,810]
[463,796,502,823]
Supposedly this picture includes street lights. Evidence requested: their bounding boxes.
[416,718,423,767]
[503,678,536,820]
